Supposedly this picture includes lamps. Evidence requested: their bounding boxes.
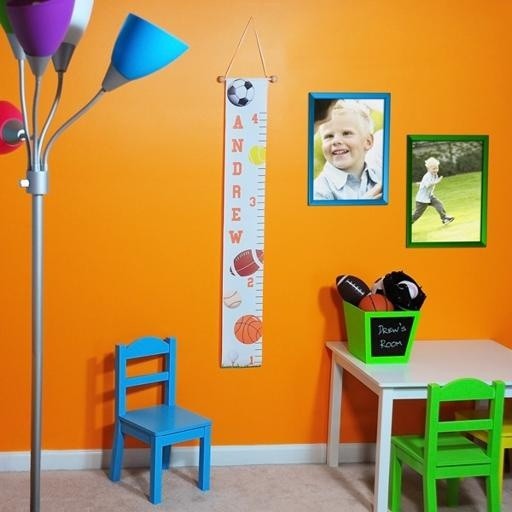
[0,1,191,511]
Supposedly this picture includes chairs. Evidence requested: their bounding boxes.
[390,377,506,511]
[111,336,215,506]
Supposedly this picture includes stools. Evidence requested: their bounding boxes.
[454,410,512,503]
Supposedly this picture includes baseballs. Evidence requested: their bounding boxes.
[249,145,266,165]
[224,290,242,309]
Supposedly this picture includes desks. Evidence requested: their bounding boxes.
[322,336,512,511]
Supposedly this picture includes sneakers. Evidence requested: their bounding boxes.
[442,216,454,225]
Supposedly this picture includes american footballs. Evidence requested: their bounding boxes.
[336,274,370,308]
[230,249,264,277]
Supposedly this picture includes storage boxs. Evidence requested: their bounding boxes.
[341,301,421,366]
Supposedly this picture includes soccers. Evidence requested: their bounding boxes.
[373,276,385,294]
[228,78,255,107]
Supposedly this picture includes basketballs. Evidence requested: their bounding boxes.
[359,294,394,311]
[234,315,262,344]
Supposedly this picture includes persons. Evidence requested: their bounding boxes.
[410,157,455,227]
[312,100,383,202]
[313,97,341,135]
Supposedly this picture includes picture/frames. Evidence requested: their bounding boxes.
[307,92,489,249]
